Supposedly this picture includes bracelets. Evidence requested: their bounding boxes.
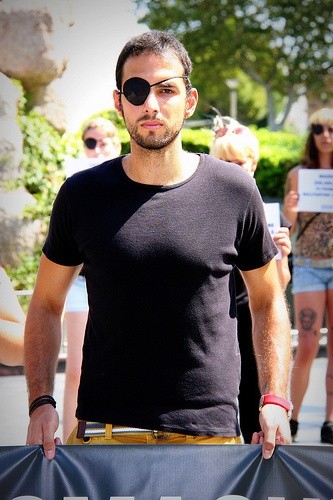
[29,395,56,418]
[259,395,293,420]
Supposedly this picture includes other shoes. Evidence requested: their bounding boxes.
[289,418,299,435]
[321,422,333,444]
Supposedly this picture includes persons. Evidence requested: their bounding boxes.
[23,30,294,460]
[283,108,333,444]
[0,265,26,367]
[83,118,122,164]
[208,109,294,444]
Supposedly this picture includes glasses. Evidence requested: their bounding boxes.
[215,127,244,138]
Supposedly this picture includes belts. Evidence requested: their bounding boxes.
[75,422,157,438]
